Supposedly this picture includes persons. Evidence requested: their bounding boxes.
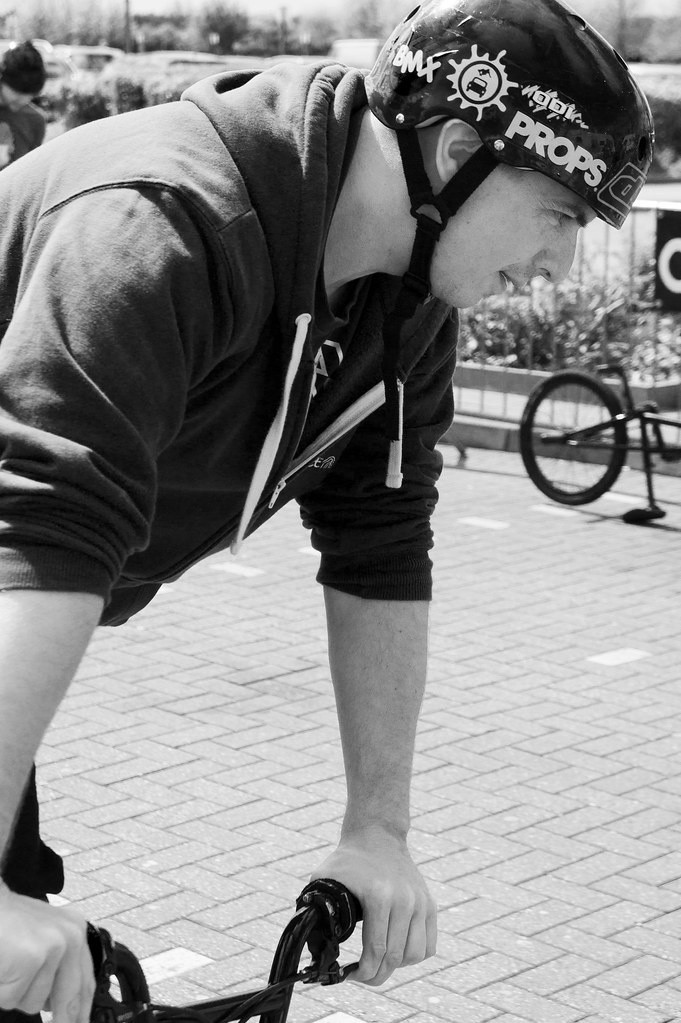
[0,0,654,1023]
[0,30,46,161]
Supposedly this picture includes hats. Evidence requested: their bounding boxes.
[0,41,45,94]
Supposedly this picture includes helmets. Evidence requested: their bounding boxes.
[364,0,655,230]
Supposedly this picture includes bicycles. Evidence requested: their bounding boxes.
[85,878,364,1023]
[516,365,681,523]
